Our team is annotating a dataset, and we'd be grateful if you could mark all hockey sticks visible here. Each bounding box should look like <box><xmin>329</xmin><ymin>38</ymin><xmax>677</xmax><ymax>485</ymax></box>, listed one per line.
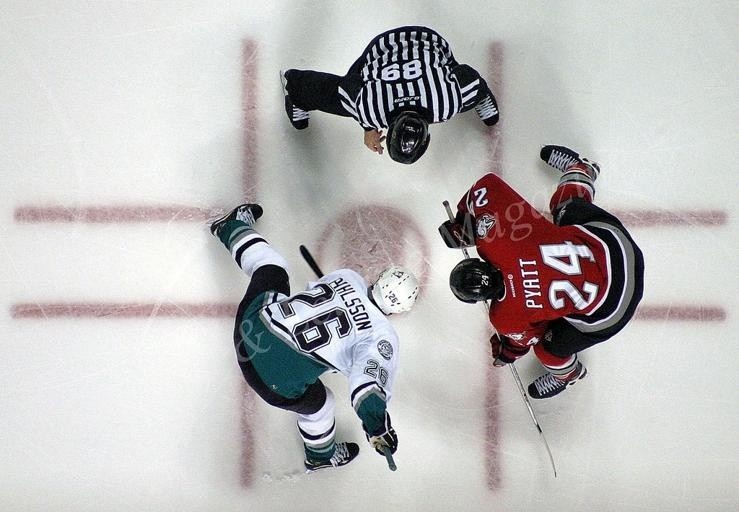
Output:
<box><xmin>443</xmin><ymin>200</ymin><xmax>556</xmax><ymax>477</ymax></box>
<box><xmin>299</xmin><ymin>245</ymin><xmax>397</xmax><ymax>471</ymax></box>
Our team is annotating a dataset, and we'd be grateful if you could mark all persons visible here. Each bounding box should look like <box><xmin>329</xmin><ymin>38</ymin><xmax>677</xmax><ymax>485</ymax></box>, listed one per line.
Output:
<box><xmin>282</xmin><ymin>25</ymin><xmax>501</xmax><ymax>164</ymax></box>
<box><xmin>438</xmin><ymin>143</ymin><xmax>646</xmax><ymax>400</ymax></box>
<box><xmin>209</xmin><ymin>202</ymin><xmax>423</xmax><ymax>474</ymax></box>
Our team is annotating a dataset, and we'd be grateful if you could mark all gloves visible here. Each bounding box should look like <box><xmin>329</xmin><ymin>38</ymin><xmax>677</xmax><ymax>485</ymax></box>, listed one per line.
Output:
<box><xmin>438</xmin><ymin>218</ymin><xmax>475</xmax><ymax>251</ymax></box>
<box><xmin>359</xmin><ymin>407</ymin><xmax>400</xmax><ymax>457</ymax></box>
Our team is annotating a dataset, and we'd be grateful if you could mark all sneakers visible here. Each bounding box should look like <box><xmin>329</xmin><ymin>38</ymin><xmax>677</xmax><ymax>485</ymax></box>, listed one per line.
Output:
<box><xmin>282</xmin><ymin>92</ymin><xmax>309</xmax><ymax>132</ymax></box>
<box><xmin>527</xmin><ymin>360</ymin><xmax>588</xmax><ymax>399</ymax></box>
<box><xmin>472</xmin><ymin>86</ymin><xmax>500</xmax><ymax>127</ymax></box>
<box><xmin>538</xmin><ymin>143</ymin><xmax>600</xmax><ymax>182</ymax></box>
<box><xmin>299</xmin><ymin>441</ymin><xmax>360</xmax><ymax>473</ymax></box>
<box><xmin>209</xmin><ymin>201</ymin><xmax>265</xmax><ymax>241</ymax></box>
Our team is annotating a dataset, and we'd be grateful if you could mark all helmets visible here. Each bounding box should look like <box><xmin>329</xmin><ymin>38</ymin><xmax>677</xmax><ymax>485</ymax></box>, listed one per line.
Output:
<box><xmin>448</xmin><ymin>256</ymin><xmax>504</xmax><ymax>305</ymax></box>
<box><xmin>381</xmin><ymin>110</ymin><xmax>431</xmax><ymax>167</ymax></box>
<box><xmin>368</xmin><ymin>262</ymin><xmax>422</xmax><ymax>315</ymax></box>
